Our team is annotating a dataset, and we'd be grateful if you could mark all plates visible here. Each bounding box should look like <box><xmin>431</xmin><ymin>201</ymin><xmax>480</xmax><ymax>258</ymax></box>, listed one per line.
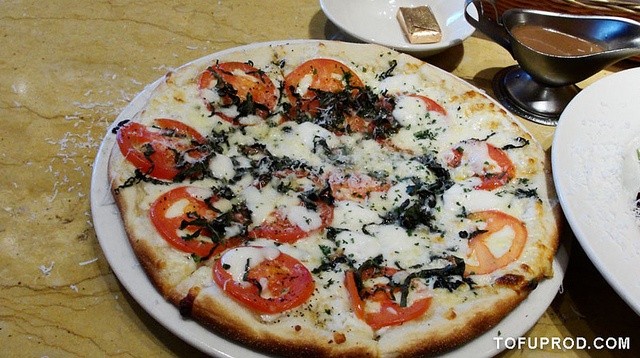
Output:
<box><xmin>551</xmin><ymin>66</ymin><xmax>640</xmax><ymax>319</ymax></box>
<box><xmin>318</xmin><ymin>0</ymin><xmax>479</xmax><ymax>57</ymax></box>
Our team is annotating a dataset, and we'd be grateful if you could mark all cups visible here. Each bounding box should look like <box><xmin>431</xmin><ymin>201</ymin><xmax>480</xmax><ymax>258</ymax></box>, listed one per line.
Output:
<box><xmin>464</xmin><ymin>0</ymin><xmax>639</xmax><ymax>126</ymax></box>
